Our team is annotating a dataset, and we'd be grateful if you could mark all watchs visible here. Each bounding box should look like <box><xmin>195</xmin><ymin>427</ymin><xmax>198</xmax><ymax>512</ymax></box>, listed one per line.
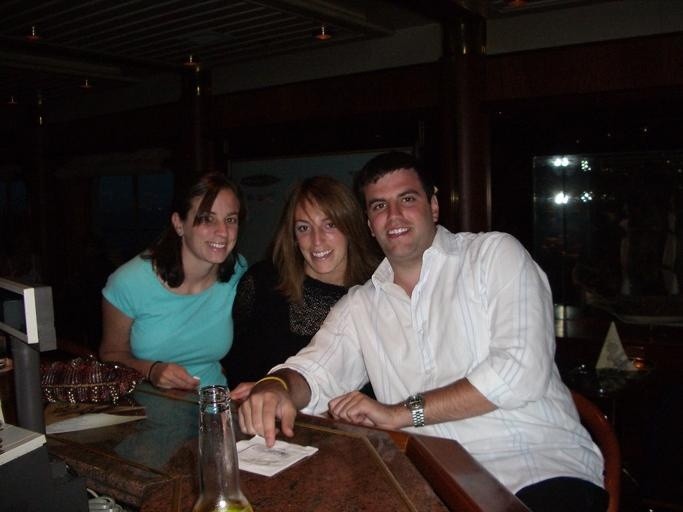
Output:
<box><xmin>407</xmin><ymin>391</ymin><xmax>427</xmax><ymax>426</ymax></box>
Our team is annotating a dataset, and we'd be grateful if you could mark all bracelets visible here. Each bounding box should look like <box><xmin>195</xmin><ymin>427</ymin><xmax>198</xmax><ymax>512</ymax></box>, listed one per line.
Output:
<box><xmin>253</xmin><ymin>375</ymin><xmax>291</xmax><ymax>392</ymax></box>
<box><xmin>146</xmin><ymin>361</ymin><xmax>162</xmax><ymax>383</ymax></box>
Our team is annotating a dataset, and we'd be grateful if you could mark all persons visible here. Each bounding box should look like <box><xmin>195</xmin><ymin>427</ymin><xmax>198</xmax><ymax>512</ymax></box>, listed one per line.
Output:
<box><xmin>97</xmin><ymin>168</ymin><xmax>250</xmax><ymax>394</ymax></box>
<box><xmin>236</xmin><ymin>151</ymin><xmax>611</xmax><ymax>511</ymax></box>
<box><xmin>218</xmin><ymin>175</ymin><xmax>380</xmax><ymax>402</ymax></box>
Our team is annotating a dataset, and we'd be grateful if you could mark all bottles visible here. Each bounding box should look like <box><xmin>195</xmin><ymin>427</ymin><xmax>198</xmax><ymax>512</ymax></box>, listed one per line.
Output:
<box><xmin>190</xmin><ymin>384</ymin><xmax>251</xmax><ymax>512</ymax></box>
